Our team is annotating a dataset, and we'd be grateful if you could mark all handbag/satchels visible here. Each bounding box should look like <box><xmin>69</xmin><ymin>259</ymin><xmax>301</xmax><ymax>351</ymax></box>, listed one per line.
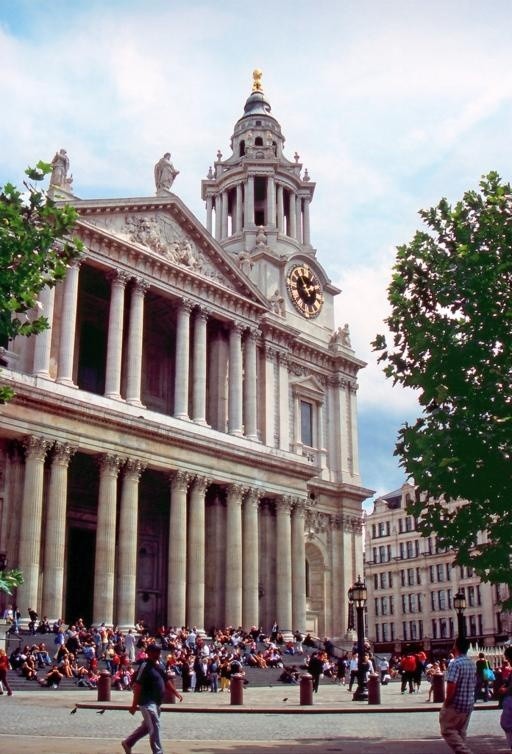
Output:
<box><xmin>483</xmin><ymin>669</ymin><xmax>496</xmax><ymax>681</ymax></box>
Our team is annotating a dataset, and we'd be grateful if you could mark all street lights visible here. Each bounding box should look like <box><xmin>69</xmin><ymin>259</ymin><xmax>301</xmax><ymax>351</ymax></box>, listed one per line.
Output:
<box><xmin>348</xmin><ymin>573</ymin><xmax>370</xmax><ymax>702</ymax></box>
<box><xmin>453</xmin><ymin>586</ymin><xmax>467</xmax><ymax>639</ymax></box>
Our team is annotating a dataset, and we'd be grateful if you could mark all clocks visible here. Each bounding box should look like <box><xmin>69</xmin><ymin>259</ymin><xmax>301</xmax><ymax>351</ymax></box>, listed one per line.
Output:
<box><xmin>287</xmin><ymin>263</ymin><xmax>325</xmax><ymax>319</ymax></box>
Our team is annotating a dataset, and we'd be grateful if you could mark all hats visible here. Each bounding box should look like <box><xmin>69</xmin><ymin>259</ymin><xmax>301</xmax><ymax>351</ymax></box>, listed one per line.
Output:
<box><xmin>479</xmin><ymin>653</ymin><xmax>484</xmax><ymax>658</ymax></box>
<box><xmin>145</xmin><ymin>645</ymin><xmax>164</xmax><ymax>652</ymax></box>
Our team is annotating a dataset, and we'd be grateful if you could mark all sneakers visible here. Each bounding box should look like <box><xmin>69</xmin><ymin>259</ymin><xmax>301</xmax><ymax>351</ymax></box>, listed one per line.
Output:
<box><xmin>122</xmin><ymin>741</ymin><xmax>131</xmax><ymax>754</ymax></box>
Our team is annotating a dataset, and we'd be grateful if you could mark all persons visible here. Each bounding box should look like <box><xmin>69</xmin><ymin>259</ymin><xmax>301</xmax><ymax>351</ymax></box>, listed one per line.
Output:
<box><xmin>121</xmin><ymin>644</ymin><xmax>186</xmax><ymax>753</ymax></box>
<box><xmin>495</xmin><ymin>644</ymin><xmax>512</xmax><ymax>751</ymax></box>
<box><xmin>437</xmin><ymin>636</ymin><xmax>478</xmax><ymax>753</ymax></box>
<box><xmin>139</xmin><ymin>216</ymin><xmax>208</xmax><ymax>274</ymax></box>
<box><xmin>0</xmin><ymin>606</ymin><xmax>512</xmax><ymax>701</ymax></box>
<box><xmin>49</xmin><ymin>148</ymin><xmax>71</xmax><ymax>186</ymax></box>
<box><xmin>158</xmin><ymin>151</ymin><xmax>178</xmax><ymax>188</ymax></box>
<box><xmin>330</xmin><ymin>323</ymin><xmax>351</xmax><ymax>347</ymax></box>
